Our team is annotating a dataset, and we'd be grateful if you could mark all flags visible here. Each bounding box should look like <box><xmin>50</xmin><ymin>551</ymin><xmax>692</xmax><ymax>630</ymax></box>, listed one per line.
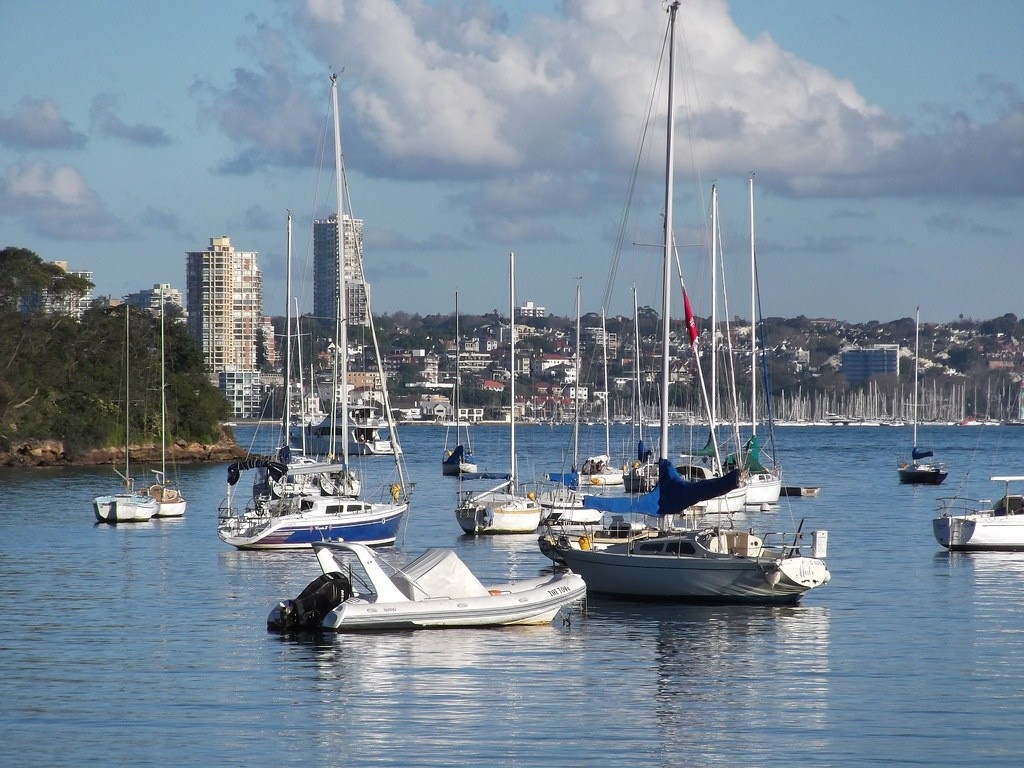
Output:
<box><xmin>684</xmin><ymin>290</ymin><xmax>699</xmax><ymax>349</ymax></box>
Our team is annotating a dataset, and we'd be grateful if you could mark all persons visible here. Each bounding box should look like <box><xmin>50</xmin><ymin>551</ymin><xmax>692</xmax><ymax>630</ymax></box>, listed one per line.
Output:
<box><xmin>581</xmin><ymin>459</ymin><xmax>606</xmax><ymax>475</ymax></box>
<box><xmin>359</xmin><ymin>434</ymin><xmax>364</xmax><ymax>442</ymax></box>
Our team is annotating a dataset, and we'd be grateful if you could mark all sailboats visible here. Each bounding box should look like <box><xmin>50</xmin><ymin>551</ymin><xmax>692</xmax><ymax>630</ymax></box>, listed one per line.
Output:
<box><xmin>443</xmin><ymin>291</ymin><xmax>476</xmax><ymax>477</ymax></box>
<box><xmin>140</xmin><ymin>286</ymin><xmax>188</xmax><ymax>517</ymax></box>
<box><xmin>624</xmin><ymin>282</ymin><xmax>661</xmax><ymax>492</ymax></box>
<box><xmin>250</xmin><ymin>209</ymin><xmax>359</xmax><ymax>513</ymax></box>
<box><xmin>931</xmin><ymin>474</ymin><xmax>1024</xmax><ymax>549</ymax></box>
<box><xmin>454</xmin><ymin>252</ymin><xmax>541</xmax><ymax>535</ymax></box>
<box><xmin>729</xmin><ymin>173</ymin><xmax>784</xmax><ymax>505</ymax></box>
<box><xmin>93</xmin><ymin>305</ymin><xmax>158</xmax><ymax>522</ymax></box>
<box><xmin>539</xmin><ymin>0</ymin><xmax>832</xmax><ymax>603</ymax></box>
<box><xmin>897</xmin><ymin>304</ymin><xmax>950</xmax><ymax>484</ymax></box>
<box><xmin>669</xmin><ymin>182</ymin><xmax>747</xmax><ymax>514</ymax></box>
<box><xmin>216</xmin><ymin>71</ymin><xmax>411</xmax><ymax>550</ymax></box>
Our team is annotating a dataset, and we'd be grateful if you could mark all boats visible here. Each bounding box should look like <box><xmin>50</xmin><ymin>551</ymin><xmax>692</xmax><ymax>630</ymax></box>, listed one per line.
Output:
<box><xmin>529</xmin><ymin>486</ymin><xmax>605</xmax><ymax>523</ymax></box>
<box><xmin>568</xmin><ymin>308</ymin><xmax>627</xmax><ymax>486</ymax></box>
<box><xmin>270</xmin><ymin>536</ymin><xmax>586</xmax><ymax>638</ymax></box>
